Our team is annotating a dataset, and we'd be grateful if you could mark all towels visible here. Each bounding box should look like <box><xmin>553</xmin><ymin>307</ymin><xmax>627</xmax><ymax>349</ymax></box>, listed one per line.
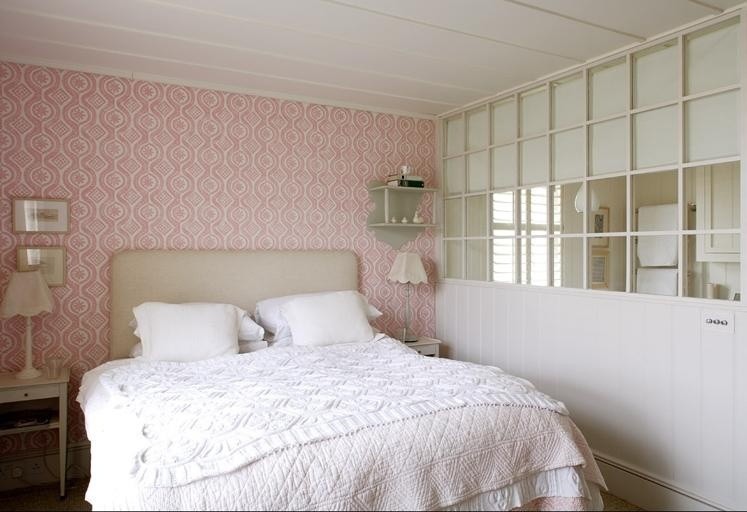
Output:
<box><xmin>637</xmin><ymin>199</ymin><xmax>681</xmax><ymax>268</ymax></box>
<box><xmin>636</xmin><ymin>268</ymin><xmax>679</xmax><ymax>297</ymax></box>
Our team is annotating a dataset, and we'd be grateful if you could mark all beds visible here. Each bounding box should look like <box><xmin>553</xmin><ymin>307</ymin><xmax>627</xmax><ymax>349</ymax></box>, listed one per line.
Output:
<box><xmin>78</xmin><ymin>249</ymin><xmax>608</xmax><ymax>512</ymax></box>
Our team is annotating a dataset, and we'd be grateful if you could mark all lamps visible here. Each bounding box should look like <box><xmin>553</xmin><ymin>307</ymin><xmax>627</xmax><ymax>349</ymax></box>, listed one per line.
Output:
<box><xmin>0</xmin><ymin>271</ymin><xmax>56</xmax><ymax>379</ymax></box>
<box><xmin>388</xmin><ymin>252</ymin><xmax>429</xmax><ymax>342</ymax></box>
<box><xmin>574</xmin><ymin>181</ymin><xmax>598</xmax><ymax>213</ymax></box>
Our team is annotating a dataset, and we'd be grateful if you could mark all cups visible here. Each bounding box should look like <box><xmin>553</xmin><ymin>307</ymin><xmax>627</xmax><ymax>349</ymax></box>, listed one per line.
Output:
<box><xmin>705</xmin><ymin>283</ymin><xmax>719</xmax><ymax>299</ymax></box>
<box><xmin>46</xmin><ymin>359</ymin><xmax>60</xmax><ymax>378</ymax></box>
<box><xmin>395</xmin><ymin>327</ymin><xmax>406</xmax><ymax>344</ymax></box>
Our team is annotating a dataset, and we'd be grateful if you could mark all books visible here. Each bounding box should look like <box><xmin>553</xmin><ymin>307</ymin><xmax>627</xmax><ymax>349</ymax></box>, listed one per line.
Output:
<box><xmin>387</xmin><ymin>174</ymin><xmax>424</xmax><ymax>188</ymax></box>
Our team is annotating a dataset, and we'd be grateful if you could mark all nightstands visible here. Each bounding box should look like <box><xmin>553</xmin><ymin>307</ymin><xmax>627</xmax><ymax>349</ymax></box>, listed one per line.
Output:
<box><xmin>404</xmin><ymin>336</ymin><xmax>441</xmax><ymax>358</ymax></box>
<box><xmin>0</xmin><ymin>368</ymin><xmax>71</xmax><ymax>498</ymax></box>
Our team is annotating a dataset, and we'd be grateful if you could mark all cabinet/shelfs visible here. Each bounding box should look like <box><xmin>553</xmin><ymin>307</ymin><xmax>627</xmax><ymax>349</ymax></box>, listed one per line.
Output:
<box><xmin>694</xmin><ymin>159</ymin><xmax>742</xmax><ymax>263</ymax></box>
<box><xmin>367</xmin><ymin>185</ymin><xmax>438</xmax><ymax>230</ymax></box>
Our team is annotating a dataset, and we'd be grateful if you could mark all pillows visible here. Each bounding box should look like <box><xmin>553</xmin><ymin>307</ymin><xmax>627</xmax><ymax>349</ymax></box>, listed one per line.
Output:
<box><xmin>254</xmin><ymin>289</ymin><xmax>384</xmax><ymax>346</ymax></box>
<box><xmin>278</xmin><ymin>293</ymin><xmax>376</xmax><ymax>343</ymax></box>
<box><xmin>135</xmin><ymin>301</ymin><xmax>241</xmax><ymax>357</ymax></box>
<box><xmin>130</xmin><ymin>299</ymin><xmax>267</xmax><ymax>359</ymax></box>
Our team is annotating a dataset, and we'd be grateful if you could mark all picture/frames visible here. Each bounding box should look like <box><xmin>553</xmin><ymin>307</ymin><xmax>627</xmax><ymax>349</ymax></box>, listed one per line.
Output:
<box><xmin>11</xmin><ymin>197</ymin><xmax>72</xmax><ymax>235</ymax></box>
<box><xmin>15</xmin><ymin>244</ymin><xmax>67</xmax><ymax>287</ymax></box>
<box><xmin>588</xmin><ymin>251</ymin><xmax>610</xmax><ymax>288</ymax></box>
<box><xmin>591</xmin><ymin>206</ymin><xmax>610</xmax><ymax>249</ymax></box>
